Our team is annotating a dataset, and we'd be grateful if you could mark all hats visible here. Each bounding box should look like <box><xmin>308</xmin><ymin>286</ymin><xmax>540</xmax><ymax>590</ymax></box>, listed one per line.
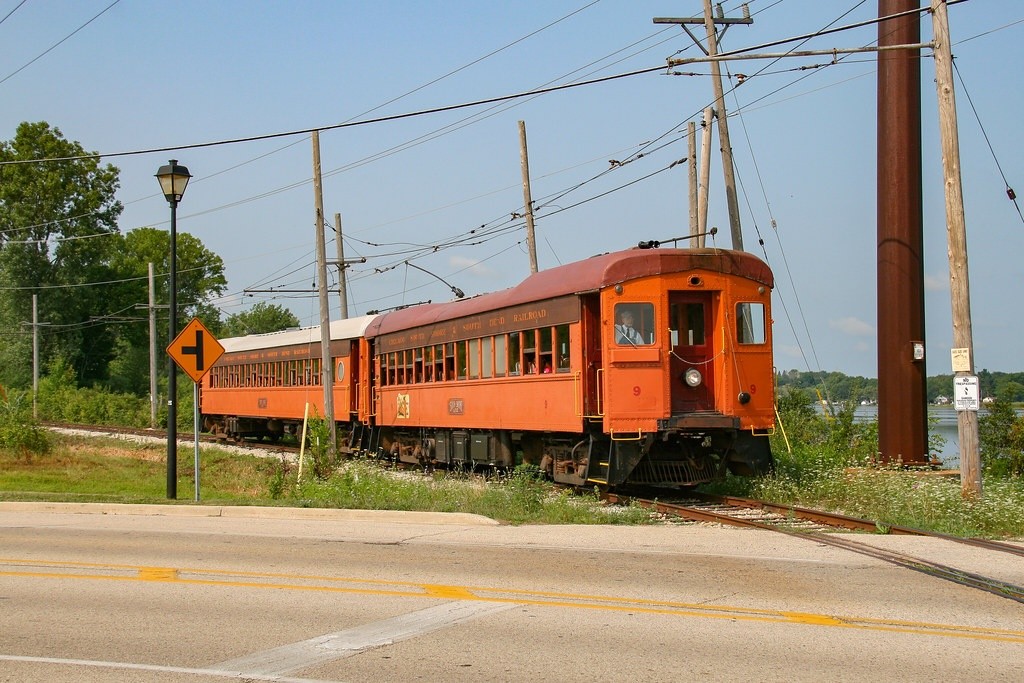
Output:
<box><xmin>621</xmin><ymin>311</ymin><xmax>635</xmax><ymax>319</ymax></box>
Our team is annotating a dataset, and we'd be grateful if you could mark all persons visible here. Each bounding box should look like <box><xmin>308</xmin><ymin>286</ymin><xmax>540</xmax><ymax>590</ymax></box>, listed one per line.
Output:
<box><xmin>614</xmin><ymin>310</ymin><xmax>645</xmax><ymax>344</ymax></box>
<box><xmin>532</xmin><ymin>356</ymin><xmax>552</xmax><ymax>374</ymax></box>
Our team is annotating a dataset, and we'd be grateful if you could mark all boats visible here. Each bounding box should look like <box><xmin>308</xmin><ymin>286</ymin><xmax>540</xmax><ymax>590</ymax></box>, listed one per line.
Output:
<box><xmin>861</xmin><ymin>400</ymin><xmax>875</xmax><ymax>406</ymax></box>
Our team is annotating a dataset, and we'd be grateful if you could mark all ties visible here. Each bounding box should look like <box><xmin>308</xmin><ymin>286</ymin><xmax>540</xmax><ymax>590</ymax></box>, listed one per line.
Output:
<box><xmin>626</xmin><ymin>328</ymin><xmax>630</xmax><ymax>337</ymax></box>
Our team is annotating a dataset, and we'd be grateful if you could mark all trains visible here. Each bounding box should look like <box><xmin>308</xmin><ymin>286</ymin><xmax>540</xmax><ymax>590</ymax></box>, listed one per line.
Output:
<box><xmin>196</xmin><ymin>229</ymin><xmax>779</xmax><ymax>494</ymax></box>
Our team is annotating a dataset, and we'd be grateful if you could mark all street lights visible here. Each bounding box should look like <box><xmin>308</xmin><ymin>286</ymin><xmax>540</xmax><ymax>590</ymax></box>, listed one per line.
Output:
<box><xmin>155</xmin><ymin>156</ymin><xmax>194</xmax><ymax>499</ymax></box>
